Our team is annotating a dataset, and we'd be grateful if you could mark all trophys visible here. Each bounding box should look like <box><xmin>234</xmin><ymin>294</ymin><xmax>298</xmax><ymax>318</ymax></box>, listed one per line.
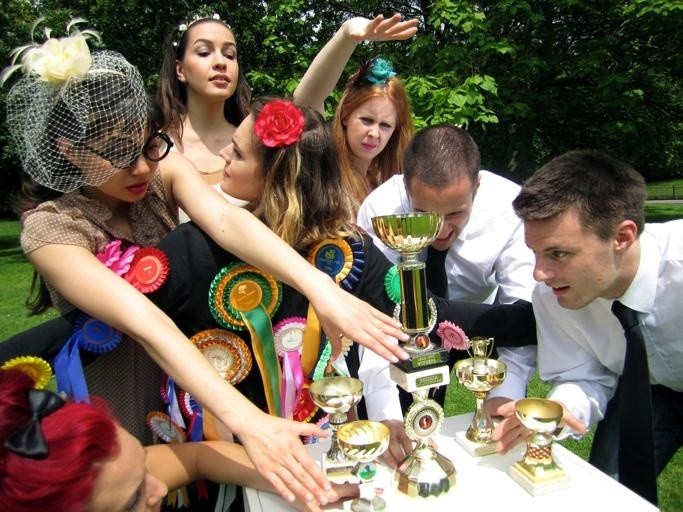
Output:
<box><xmin>338</xmin><ymin>420</ymin><xmax>389</xmax><ymax>511</ymax></box>
<box><xmin>309</xmin><ymin>377</ymin><xmax>363</xmax><ymax>429</ymax></box>
<box><xmin>453</xmin><ymin>335</ymin><xmax>507</xmax><ymax>456</ymax></box>
<box><xmin>371</xmin><ymin>213</ymin><xmax>451</xmax><ymax>394</ymax></box>
<box><xmin>395</xmin><ymin>400</ymin><xmax>456</xmax><ymax>497</ymax></box>
<box><xmin>510</xmin><ymin>398</ymin><xmax>567</xmax><ymax>496</ymax></box>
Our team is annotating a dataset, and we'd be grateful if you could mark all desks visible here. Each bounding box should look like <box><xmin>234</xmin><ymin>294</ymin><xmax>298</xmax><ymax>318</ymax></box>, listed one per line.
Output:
<box><xmin>241</xmin><ymin>410</ymin><xmax>660</xmax><ymax>511</ymax></box>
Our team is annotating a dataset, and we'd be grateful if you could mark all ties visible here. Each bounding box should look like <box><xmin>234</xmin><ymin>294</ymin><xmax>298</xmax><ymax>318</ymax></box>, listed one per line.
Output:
<box><xmin>611</xmin><ymin>301</ymin><xmax>658</xmax><ymax>506</ymax></box>
<box><xmin>425</xmin><ymin>245</ymin><xmax>451</xmax><ymax>298</ymax></box>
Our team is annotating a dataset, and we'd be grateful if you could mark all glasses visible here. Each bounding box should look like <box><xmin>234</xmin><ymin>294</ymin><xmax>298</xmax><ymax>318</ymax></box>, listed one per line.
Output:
<box><xmin>75</xmin><ymin>133</ymin><xmax>174</xmax><ymax>168</ymax></box>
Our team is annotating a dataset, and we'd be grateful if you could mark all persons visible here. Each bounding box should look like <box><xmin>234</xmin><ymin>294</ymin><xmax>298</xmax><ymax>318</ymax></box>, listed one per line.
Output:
<box><xmin>358</xmin><ymin>122</ymin><xmax>538</xmax><ymax>423</ymax></box>
<box><xmin>331</xmin><ymin>55</ymin><xmax>414</xmax><ymax>209</ymax></box>
<box><xmin>0</xmin><ymin>365</ymin><xmax>360</xmax><ymax>512</ymax></box>
<box><xmin>157</xmin><ymin>12</ymin><xmax>418</xmax><ymax>222</ymax></box>
<box><xmin>493</xmin><ymin>149</ymin><xmax>682</xmax><ymax>485</ymax></box>
<box><xmin>0</xmin><ymin>93</ymin><xmax>538</xmax><ymax>433</ymax></box>
<box><xmin>0</xmin><ymin>17</ymin><xmax>410</xmax><ymax>506</ymax></box>
